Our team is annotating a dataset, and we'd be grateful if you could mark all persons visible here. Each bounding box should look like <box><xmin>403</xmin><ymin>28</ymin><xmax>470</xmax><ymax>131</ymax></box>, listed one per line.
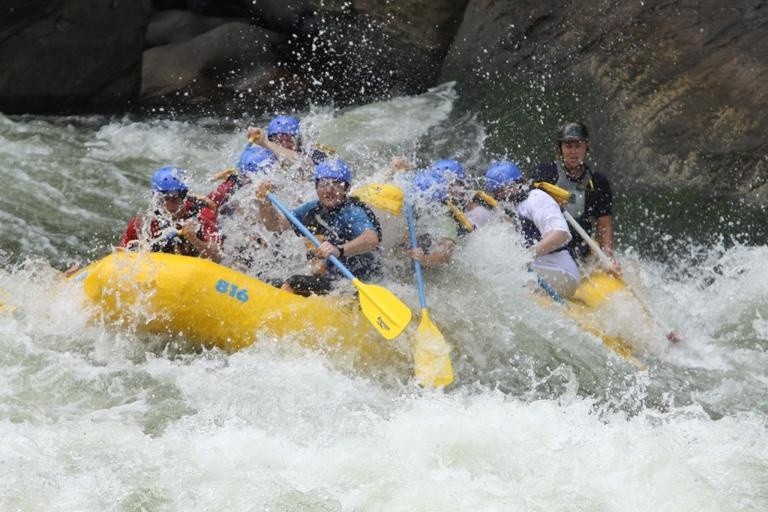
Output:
<box><xmin>370</xmin><ymin>120</ymin><xmax>489</xmax><ymax>284</ymax></box>
<box><xmin>484</xmin><ymin>161</ymin><xmax>579</xmax><ymax>300</ymax></box>
<box><xmin>120</xmin><ymin>115</ymin><xmax>383</xmax><ymax>299</ymax></box>
<box><xmin>523</xmin><ymin>122</ymin><xmax>623</xmax><ymax>281</ymax></box>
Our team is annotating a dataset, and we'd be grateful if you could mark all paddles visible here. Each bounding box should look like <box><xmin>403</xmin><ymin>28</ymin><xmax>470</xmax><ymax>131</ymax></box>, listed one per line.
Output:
<box><xmin>264</xmin><ymin>189</ymin><xmax>411</xmax><ymax>340</ymax></box>
<box><xmin>402</xmin><ymin>169</ymin><xmax>453</xmax><ymax>389</ymax></box>
<box><xmin>473</xmin><ymin>192</ymin><xmax>631</xmax><ymax>361</ymax></box>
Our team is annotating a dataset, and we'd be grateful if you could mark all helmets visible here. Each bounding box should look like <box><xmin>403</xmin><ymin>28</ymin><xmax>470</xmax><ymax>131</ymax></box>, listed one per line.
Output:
<box><xmin>268</xmin><ymin>115</ymin><xmax>301</xmax><ymax>139</ymax></box>
<box><xmin>413</xmin><ymin>172</ymin><xmax>449</xmax><ymax>204</ymax></box>
<box><xmin>433</xmin><ymin>159</ymin><xmax>466</xmax><ymax>185</ymax></box>
<box><xmin>314</xmin><ymin>158</ymin><xmax>351</xmax><ymax>185</ymax></box>
<box><xmin>238</xmin><ymin>147</ymin><xmax>279</xmax><ymax>177</ymax></box>
<box><xmin>485</xmin><ymin>160</ymin><xmax>522</xmax><ymax>191</ymax></box>
<box><xmin>152</xmin><ymin>167</ymin><xmax>190</xmax><ymax>193</ymax></box>
<box><xmin>555</xmin><ymin>121</ymin><xmax>589</xmax><ymax>152</ymax></box>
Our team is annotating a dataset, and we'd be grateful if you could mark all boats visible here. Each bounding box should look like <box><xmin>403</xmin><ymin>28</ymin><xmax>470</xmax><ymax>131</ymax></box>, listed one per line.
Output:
<box><xmin>58</xmin><ymin>181</ymin><xmax>671</xmax><ymax>390</ymax></box>
